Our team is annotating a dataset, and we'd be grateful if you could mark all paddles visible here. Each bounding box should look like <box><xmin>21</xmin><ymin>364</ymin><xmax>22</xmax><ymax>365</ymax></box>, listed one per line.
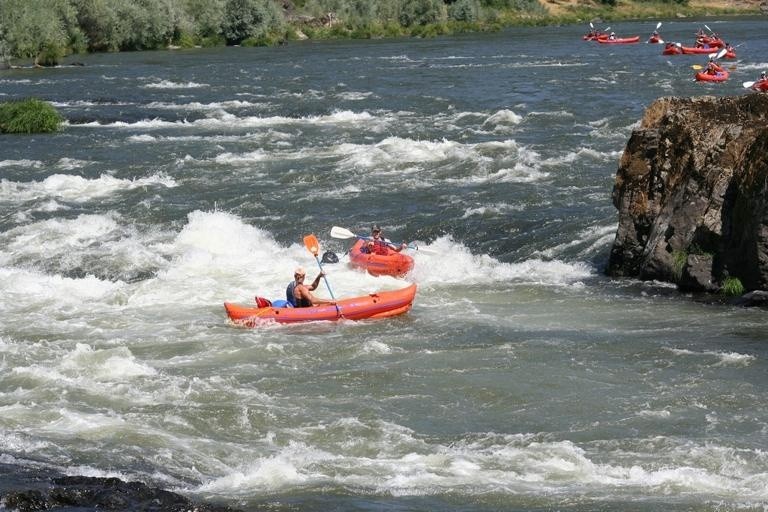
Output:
<box><xmin>590</xmin><ymin>23</ymin><xmax>594</xmax><ymax>29</ymax></box>
<box><xmin>604</xmin><ymin>27</ymin><xmax>611</xmax><ymax>32</ymax></box>
<box><xmin>303</xmin><ymin>234</ymin><xmax>344</xmax><ymax>319</ymax></box>
<box><xmin>330</xmin><ymin>226</ymin><xmax>438</xmax><ymax>256</ymax></box>
<box><xmin>705</xmin><ymin>25</ymin><xmax>714</xmax><ymax>34</ymax></box>
<box><xmin>708</xmin><ymin>44</ymin><xmax>743</xmax><ymax>59</ymax></box>
<box><xmin>643</xmin><ymin>22</ymin><xmax>662</xmax><ymax>43</ymax></box>
<box><xmin>693</xmin><ymin>64</ymin><xmax>737</xmax><ymax>71</ymax></box>
<box><xmin>692</xmin><ymin>48</ymin><xmax>727</xmax><ymax>82</ymax></box>
<box><xmin>742</xmin><ymin>81</ymin><xmax>756</xmax><ymax>89</ymax></box>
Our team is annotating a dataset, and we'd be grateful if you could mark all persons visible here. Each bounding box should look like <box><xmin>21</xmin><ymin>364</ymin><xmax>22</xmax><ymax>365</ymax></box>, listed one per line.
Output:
<box><xmin>587</xmin><ymin>28</ymin><xmax>734</xmax><ymax>75</ymax></box>
<box><xmin>362</xmin><ymin>223</ymin><xmax>406</xmax><ymax>254</ymax></box>
<box><xmin>286</xmin><ymin>268</ymin><xmax>335</xmax><ymax>306</ymax></box>
<box><xmin>760</xmin><ymin>71</ymin><xmax>768</xmax><ymax>81</ymax></box>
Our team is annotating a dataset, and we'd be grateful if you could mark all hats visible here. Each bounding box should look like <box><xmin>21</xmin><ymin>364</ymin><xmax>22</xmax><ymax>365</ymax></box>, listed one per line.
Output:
<box><xmin>295</xmin><ymin>266</ymin><xmax>307</xmax><ymax>274</ymax></box>
<box><xmin>372</xmin><ymin>224</ymin><xmax>380</xmax><ymax>232</ymax></box>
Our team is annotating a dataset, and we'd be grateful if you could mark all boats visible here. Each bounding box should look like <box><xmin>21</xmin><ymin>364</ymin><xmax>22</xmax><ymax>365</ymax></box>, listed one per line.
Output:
<box><xmin>650</xmin><ymin>34</ymin><xmax>661</xmax><ymax>43</ymax></box>
<box><xmin>349</xmin><ymin>237</ymin><xmax>416</xmax><ymax>278</ymax></box>
<box><xmin>598</xmin><ymin>36</ymin><xmax>640</xmax><ymax>44</ymax></box>
<box><xmin>695</xmin><ymin>71</ymin><xmax>730</xmax><ymax>82</ymax></box>
<box><xmin>748</xmin><ymin>79</ymin><xmax>768</xmax><ymax>93</ymax></box>
<box><xmin>224</xmin><ymin>281</ymin><xmax>418</xmax><ymax>329</ymax></box>
<box><xmin>663</xmin><ymin>46</ymin><xmax>681</xmax><ymax>56</ymax></box>
<box><xmin>724</xmin><ymin>50</ymin><xmax>736</xmax><ymax>59</ymax></box>
<box><xmin>681</xmin><ymin>38</ymin><xmax>726</xmax><ymax>55</ymax></box>
<box><xmin>582</xmin><ymin>33</ymin><xmax>609</xmax><ymax>42</ymax></box>
